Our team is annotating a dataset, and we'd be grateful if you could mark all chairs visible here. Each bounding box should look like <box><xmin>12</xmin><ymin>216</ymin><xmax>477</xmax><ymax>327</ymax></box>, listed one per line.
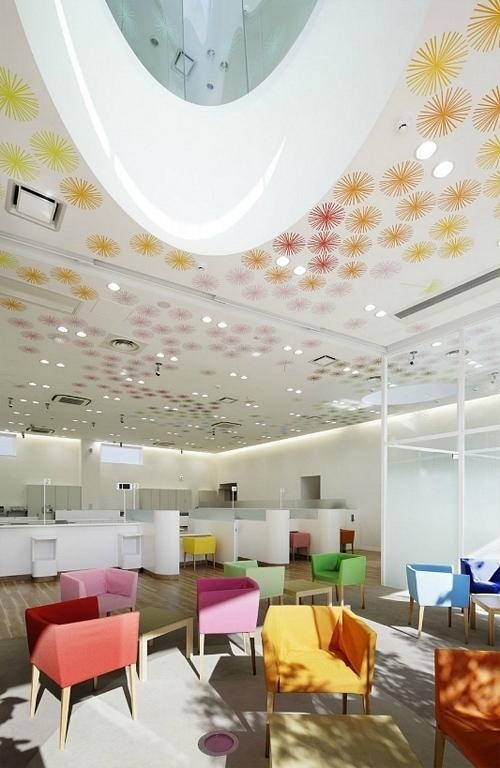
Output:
<box><xmin>196</xmin><ymin>577</ymin><xmax>260</xmax><ymax>656</ymax></box>
<box><xmin>24</xmin><ymin>596</ymin><xmax>140</xmax><ymax>751</ymax></box>
<box><xmin>224</xmin><ymin>560</ymin><xmax>285</xmax><ymax>605</ymax></box>
<box><xmin>460</xmin><ymin>558</ymin><xmax>500</xmax><ymax>593</ymax></box>
<box><xmin>434</xmin><ymin>647</ymin><xmax>500</xmax><ymax>768</ymax></box>
<box><xmin>406</xmin><ymin>563</ymin><xmax>471</xmax><ymax>644</ymax></box>
<box><xmin>261</xmin><ymin>605</ymin><xmax>377</xmax><ymax>715</ymax></box>
<box><xmin>60</xmin><ymin>568</ymin><xmax>138</xmax><ymax>616</ymax></box>
<box><xmin>311</xmin><ymin>552</ymin><xmax>366</xmax><ymax>609</ymax></box>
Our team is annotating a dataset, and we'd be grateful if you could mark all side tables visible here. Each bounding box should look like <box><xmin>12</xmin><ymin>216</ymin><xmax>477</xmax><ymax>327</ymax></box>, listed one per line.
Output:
<box><xmin>136</xmin><ymin>607</ymin><xmax>195</xmax><ymax>682</ymax></box>
<box><xmin>283</xmin><ymin>579</ymin><xmax>332</xmax><ymax>606</ymax></box>
<box><xmin>265</xmin><ymin>713</ymin><xmax>424</xmax><ymax>768</ymax></box>
<box><xmin>470</xmin><ymin>594</ymin><xmax>500</xmax><ymax>646</ymax></box>
